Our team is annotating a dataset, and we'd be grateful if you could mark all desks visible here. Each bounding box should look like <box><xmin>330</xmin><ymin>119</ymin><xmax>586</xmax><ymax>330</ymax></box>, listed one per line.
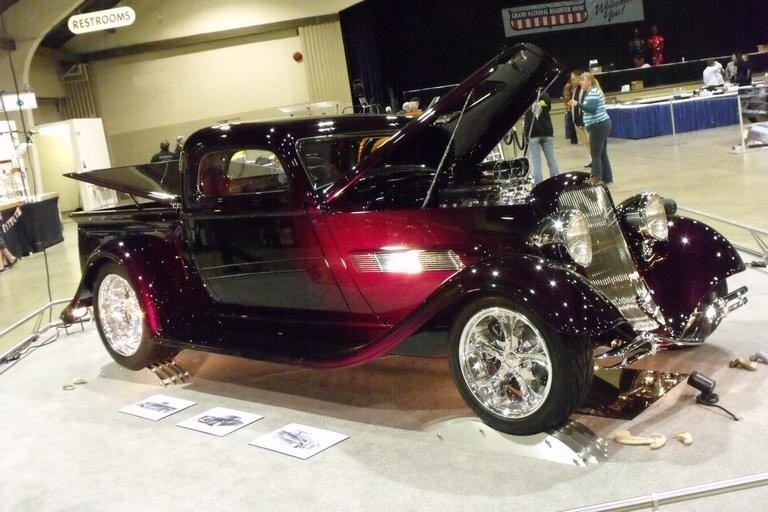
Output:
<box><xmin>593</xmin><ymin>50</ymin><xmax>768</xmax><ymax>139</ymax></box>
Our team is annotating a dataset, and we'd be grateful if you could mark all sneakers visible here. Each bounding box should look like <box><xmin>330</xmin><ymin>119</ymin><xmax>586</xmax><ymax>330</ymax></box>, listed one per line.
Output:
<box><xmin>582</xmin><ymin>175</ymin><xmax>613</xmax><ymax>186</ymax></box>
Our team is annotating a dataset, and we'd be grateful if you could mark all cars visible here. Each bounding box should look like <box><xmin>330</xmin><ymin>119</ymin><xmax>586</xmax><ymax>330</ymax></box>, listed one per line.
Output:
<box><xmin>56</xmin><ymin>38</ymin><xmax>749</xmax><ymax>438</ymax></box>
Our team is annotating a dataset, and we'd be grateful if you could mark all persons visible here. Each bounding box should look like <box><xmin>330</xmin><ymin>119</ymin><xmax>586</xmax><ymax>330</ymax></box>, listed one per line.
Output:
<box><xmin>737</xmin><ymin>53</ymin><xmax>753</xmax><ymax>86</ymax></box>
<box><xmin>571</xmin><ymin>70</ymin><xmax>593</xmax><ymax>167</ymax></box>
<box><xmin>524</xmin><ymin>92</ymin><xmax>560</xmax><ymax>187</ymax></box>
<box><xmin>395</xmin><ymin>97</ymin><xmax>422</xmax><ymax>116</ymax></box>
<box><xmin>567</xmin><ymin>72</ymin><xmax>613</xmax><ymax>184</ymax></box>
<box><xmin>150</xmin><ymin>140</ymin><xmax>179</xmax><ymax>162</ymax></box>
<box><xmin>563</xmin><ymin>78</ymin><xmax>578</xmax><ymax>138</ymax></box>
<box><xmin>633</xmin><ymin>54</ymin><xmax>652</xmax><ymax>68</ymax></box>
<box><xmin>174</xmin><ymin>135</ymin><xmax>184</xmax><ymax>155</ymax></box>
<box><xmin>726</xmin><ymin>54</ymin><xmax>741</xmax><ymax>83</ymax></box>
<box><xmin>703</xmin><ymin>59</ymin><xmax>726</xmax><ymax>89</ymax></box>
